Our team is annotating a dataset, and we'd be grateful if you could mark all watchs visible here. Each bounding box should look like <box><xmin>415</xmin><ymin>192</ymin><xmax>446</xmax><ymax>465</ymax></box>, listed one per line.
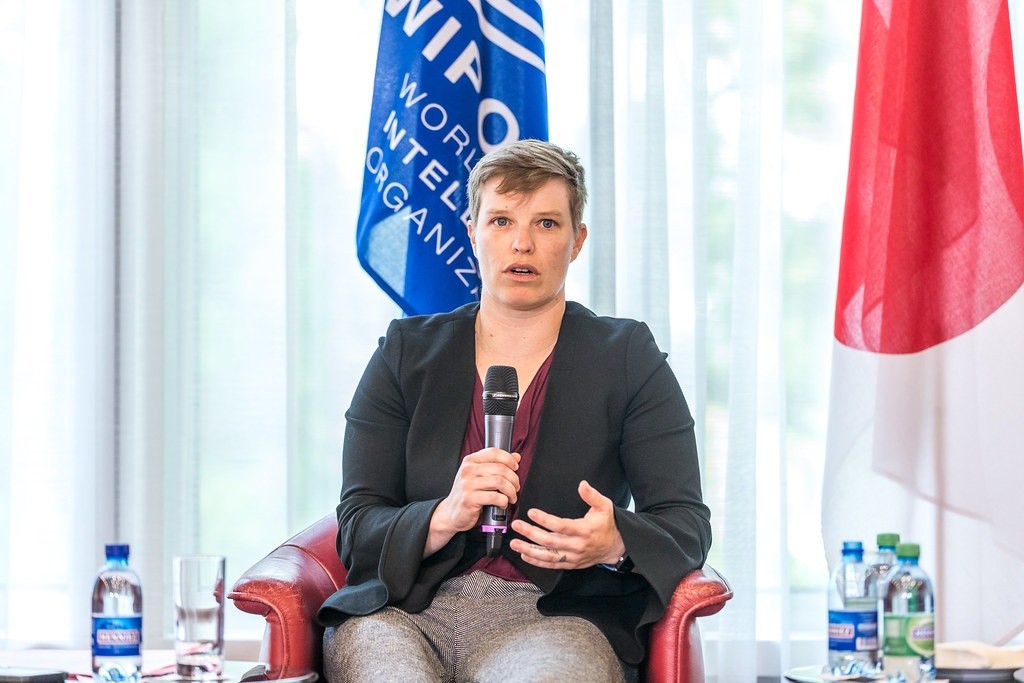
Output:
<box><xmin>597</xmin><ymin>551</ymin><xmax>634</xmax><ymax>574</ymax></box>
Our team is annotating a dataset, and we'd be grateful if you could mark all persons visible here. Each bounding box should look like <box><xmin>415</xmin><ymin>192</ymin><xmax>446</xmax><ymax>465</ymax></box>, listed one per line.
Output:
<box><xmin>315</xmin><ymin>140</ymin><xmax>712</xmax><ymax>682</ymax></box>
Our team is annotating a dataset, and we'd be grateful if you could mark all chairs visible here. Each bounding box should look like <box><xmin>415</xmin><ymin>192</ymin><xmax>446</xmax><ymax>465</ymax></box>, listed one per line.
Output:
<box><xmin>231</xmin><ymin>522</ymin><xmax>736</xmax><ymax>683</ymax></box>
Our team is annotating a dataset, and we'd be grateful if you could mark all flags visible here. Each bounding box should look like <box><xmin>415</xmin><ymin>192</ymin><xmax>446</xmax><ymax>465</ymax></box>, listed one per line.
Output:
<box><xmin>821</xmin><ymin>0</ymin><xmax>1024</xmax><ymax>645</ymax></box>
<box><xmin>357</xmin><ymin>0</ymin><xmax>550</xmax><ymax>316</ymax></box>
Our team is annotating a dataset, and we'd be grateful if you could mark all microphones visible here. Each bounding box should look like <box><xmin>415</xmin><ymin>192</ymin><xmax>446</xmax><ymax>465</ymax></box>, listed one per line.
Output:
<box><xmin>477</xmin><ymin>366</ymin><xmax>520</xmax><ymax>556</ymax></box>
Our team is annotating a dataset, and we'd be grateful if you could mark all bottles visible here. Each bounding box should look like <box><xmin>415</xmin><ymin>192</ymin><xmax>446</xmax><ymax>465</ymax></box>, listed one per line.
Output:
<box><xmin>870</xmin><ymin>533</ymin><xmax>901</xmax><ymax>601</ymax></box>
<box><xmin>90</xmin><ymin>544</ymin><xmax>144</xmax><ymax>683</ymax></box>
<box><xmin>825</xmin><ymin>539</ymin><xmax>883</xmax><ymax>679</ymax></box>
<box><xmin>879</xmin><ymin>543</ymin><xmax>937</xmax><ymax>682</ymax></box>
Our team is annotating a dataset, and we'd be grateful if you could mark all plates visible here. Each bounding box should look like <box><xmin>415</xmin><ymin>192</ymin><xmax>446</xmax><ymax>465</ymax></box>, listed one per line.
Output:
<box><xmin>929</xmin><ymin>641</ymin><xmax>1024</xmax><ymax>683</ymax></box>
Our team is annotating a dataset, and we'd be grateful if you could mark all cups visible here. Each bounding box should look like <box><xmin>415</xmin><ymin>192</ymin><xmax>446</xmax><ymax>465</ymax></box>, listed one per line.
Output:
<box><xmin>170</xmin><ymin>555</ymin><xmax>226</xmax><ymax>678</ymax></box>
